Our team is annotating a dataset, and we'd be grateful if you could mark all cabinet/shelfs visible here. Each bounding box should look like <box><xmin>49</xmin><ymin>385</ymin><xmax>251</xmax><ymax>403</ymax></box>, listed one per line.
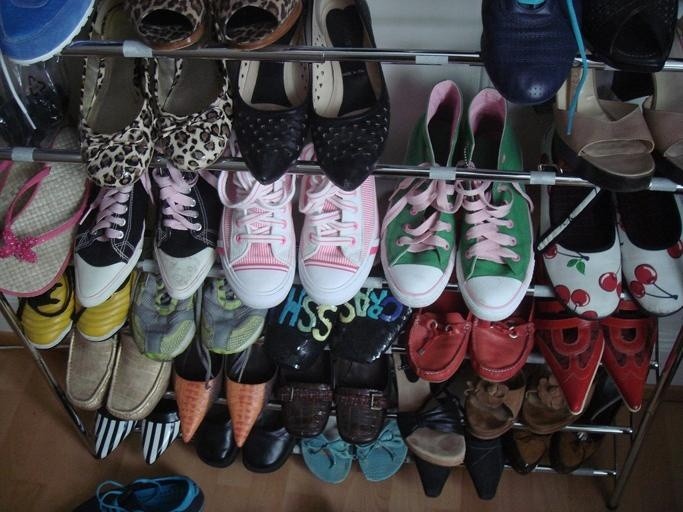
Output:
<box><xmin>0</xmin><ymin>3</ymin><xmax>683</xmax><ymax>509</ymax></box>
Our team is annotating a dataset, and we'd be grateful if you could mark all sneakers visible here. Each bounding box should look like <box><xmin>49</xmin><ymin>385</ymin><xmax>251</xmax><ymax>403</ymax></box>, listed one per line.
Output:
<box><xmin>69</xmin><ymin>475</ymin><xmax>204</xmax><ymax>510</ymax></box>
<box><xmin>0</xmin><ymin>0</ymin><xmax>683</xmax><ymax>500</ymax></box>
